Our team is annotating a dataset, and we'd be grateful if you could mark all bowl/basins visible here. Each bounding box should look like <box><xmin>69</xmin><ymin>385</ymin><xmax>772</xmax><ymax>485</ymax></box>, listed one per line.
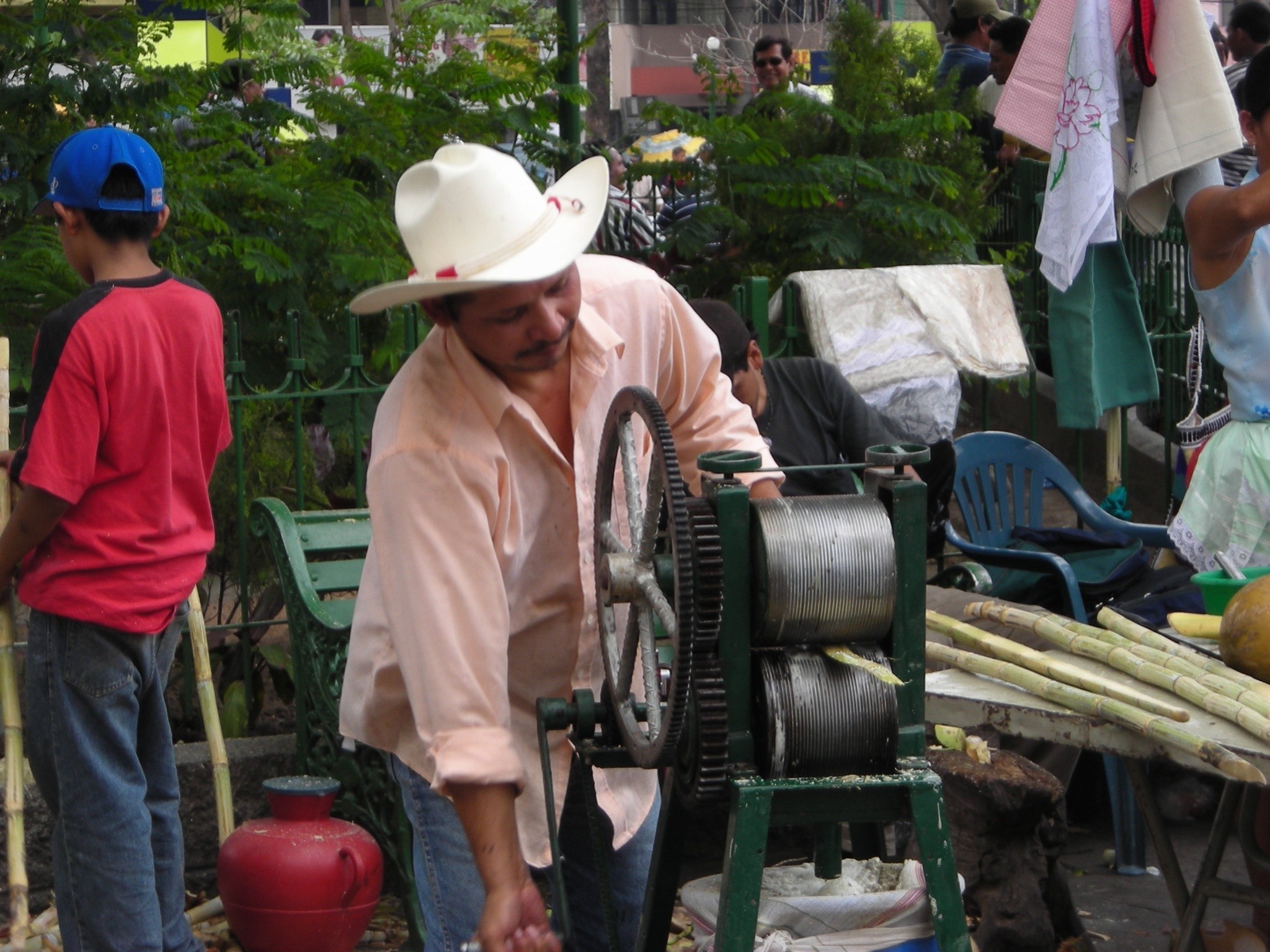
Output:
<box><xmin>1190</xmin><ymin>566</ymin><xmax>1269</xmax><ymax>616</ymax></box>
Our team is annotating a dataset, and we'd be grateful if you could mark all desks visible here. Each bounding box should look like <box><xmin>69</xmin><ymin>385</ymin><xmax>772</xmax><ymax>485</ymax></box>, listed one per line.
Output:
<box><xmin>925</xmin><ymin>626</ymin><xmax>1270</xmax><ymax>952</ymax></box>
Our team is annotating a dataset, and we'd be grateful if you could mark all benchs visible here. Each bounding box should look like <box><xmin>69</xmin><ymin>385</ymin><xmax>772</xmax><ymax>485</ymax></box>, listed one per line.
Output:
<box><xmin>252</xmin><ymin>466</ymin><xmax>996</xmax><ymax>952</ymax></box>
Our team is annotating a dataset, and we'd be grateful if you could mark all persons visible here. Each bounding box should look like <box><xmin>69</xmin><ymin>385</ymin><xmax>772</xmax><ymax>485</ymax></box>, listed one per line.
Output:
<box><xmin>0</xmin><ymin>125</ymin><xmax>232</xmax><ymax>952</ymax></box>
<box><xmin>931</xmin><ymin>0</ymin><xmax>1016</xmax><ymax>109</ymax></box>
<box><xmin>577</xmin><ymin>139</ymin><xmax>738</xmax><ymax>279</ymax></box>
<box><xmin>1212</xmin><ymin>0</ymin><xmax>1270</xmax><ymax>187</ymax></box>
<box><xmin>148</xmin><ymin>56</ymin><xmax>281</xmax><ymax>167</ymax></box>
<box><xmin>970</xmin><ymin>15</ymin><xmax>1052</xmax><ymax>163</ymax></box>
<box><xmin>749</xmin><ymin>34</ymin><xmax>830</xmax><ymax>107</ymax></box>
<box><xmin>689</xmin><ymin>295</ymin><xmax>922</xmax><ymax>496</ymax></box>
<box><xmin>339</xmin><ymin>140</ymin><xmax>790</xmax><ymax>951</ymax></box>
<box><xmin>309</xmin><ymin>28</ymin><xmax>345</xmax><ymax>86</ymax></box>
<box><xmin>439</xmin><ymin>28</ymin><xmax>477</xmax><ymax>60</ymax></box>
<box><xmin>1160</xmin><ymin>42</ymin><xmax>1269</xmax><ymax>572</ymax></box>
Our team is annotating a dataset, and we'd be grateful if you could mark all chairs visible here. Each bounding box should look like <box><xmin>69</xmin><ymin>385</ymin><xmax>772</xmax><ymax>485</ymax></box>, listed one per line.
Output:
<box><xmin>940</xmin><ymin>430</ymin><xmax>1206</xmax><ymax>877</ymax></box>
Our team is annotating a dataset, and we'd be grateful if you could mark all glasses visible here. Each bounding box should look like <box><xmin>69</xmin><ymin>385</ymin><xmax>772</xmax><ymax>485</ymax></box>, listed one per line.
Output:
<box><xmin>754</xmin><ymin>58</ymin><xmax>783</xmax><ymax>67</ymax></box>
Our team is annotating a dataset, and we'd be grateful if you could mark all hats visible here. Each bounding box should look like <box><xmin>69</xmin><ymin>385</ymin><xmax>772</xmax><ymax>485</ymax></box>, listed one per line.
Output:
<box><xmin>32</xmin><ymin>124</ymin><xmax>164</xmax><ymax>214</ymax></box>
<box><xmin>351</xmin><ymin>143</ymin><xmax>610</xmax><ymax>319</ymax></box>
<box><xmin>699</xmin><ymin>142</ymin><xmax>714</xmax><ymax>152</ymax></box>
<box><xmin>944</xmin><ymin>0</ymin><xmax>1015</xmax><ymax>35</ymax></box>
